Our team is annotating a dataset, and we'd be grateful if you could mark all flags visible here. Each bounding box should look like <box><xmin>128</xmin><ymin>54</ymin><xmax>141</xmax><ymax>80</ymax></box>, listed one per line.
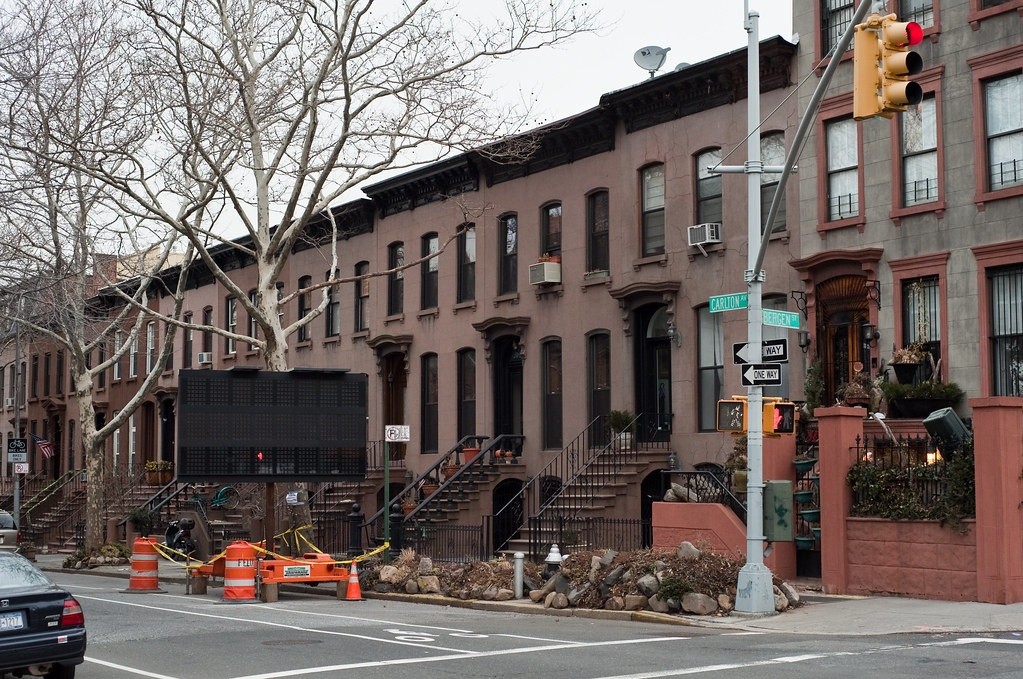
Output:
<box><xmin>29</xmin><ymin>433</ymin><xmax>54</xmax><ymax>458</ymax></box>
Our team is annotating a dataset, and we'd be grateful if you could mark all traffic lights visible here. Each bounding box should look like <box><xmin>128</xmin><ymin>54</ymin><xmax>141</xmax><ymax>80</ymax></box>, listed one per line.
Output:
<box><xmin>764</xmin><ymin>402</ymin><xmax>796</xmax><ymax>435</ymax></box>
<box><xmin>853</xmin><ymin>31</ymin><xmax>896</xmax><ymax>121</ymax></box>
<box><xmin>716</xmin><ymin>400</ymin><xmax>748</xmax><ymax>432</ymax></box>
<box><xmin>881</xmin><ymin>18</ymin><xmax>924</xmax><ymax>111</ymax></box>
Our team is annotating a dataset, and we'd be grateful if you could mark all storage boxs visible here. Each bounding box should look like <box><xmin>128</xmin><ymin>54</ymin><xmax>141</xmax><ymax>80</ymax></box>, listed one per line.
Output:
<box><xmin>180</xmin><ymin>518</ymin><xmax>195</xmax><ymax>530</ymax></box>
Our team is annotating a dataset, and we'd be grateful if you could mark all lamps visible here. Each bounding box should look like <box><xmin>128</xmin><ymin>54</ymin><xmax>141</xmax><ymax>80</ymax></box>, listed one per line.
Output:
<box><xmin>796</xmin><ymin>331</ymin><xmax>810</xmax><ymax>353</ymax></box>
<box><xmin>667</xmin><ymin>322</ymin><xmax>681</xmax><ymax>347</ymax></box>
<box><xmin>669</xmin><ymin>451</ymin><xmax>680</xmax><ymax>471</ymax></box>
<box><xmin>862</xmin><ymin>324</ymin><xmax>880</xmax><ymax>348</ymax></box>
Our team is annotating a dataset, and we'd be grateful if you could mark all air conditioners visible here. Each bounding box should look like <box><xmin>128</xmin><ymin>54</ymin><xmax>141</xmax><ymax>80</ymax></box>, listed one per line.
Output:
<box><xmin>5</xmin><ymin>398</ymin><xmax>14</xmax><ymax>407</ymax></box>
<box><xmin>198</xmin><ymin>352</ymin><xmax>212</xmax><ymax>364</ymax></box>
<box><xmin>529</xmin><ymin>262</ymin><xmax>561</xmax><ymax>286</ymax></box>
<box><xmin>687</xmin><ymin>223</ymin><xmax>723</xmax><ymax>246</ymax></box>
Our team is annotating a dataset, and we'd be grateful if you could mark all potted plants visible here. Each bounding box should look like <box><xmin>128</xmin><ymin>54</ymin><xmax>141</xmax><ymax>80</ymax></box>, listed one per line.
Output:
<box><xmin>130</xmin><ymin>506</ymin><xmax>149</xmax><ymax>551</ymax></box>
<box><xmin>884</xmin><ymin>379</ymin><xmax>966</xmax><ymax>419</ymax></box>
<box><xmin>421</xmin><ymin>478</ymin><xmax>439</xmax><ymax>498</ymax></box>
<box><xmin>144</xmin><ymin>460</ymin><xmax>174</xmax><ymax>485</ymax></box>
<box><xmin>604</xmin><ymin>409</ymin><xmax>634</xmax><ymax>450</ymax></box>
<box><xmin>20</xmin><ymin>543</ymin><xmax>37</xmax><ymax>559</ymax></box>
<box><xmin>804</xmin><ymin>359</ymin><xmax>825</xmax><ymax>442</ymax></box>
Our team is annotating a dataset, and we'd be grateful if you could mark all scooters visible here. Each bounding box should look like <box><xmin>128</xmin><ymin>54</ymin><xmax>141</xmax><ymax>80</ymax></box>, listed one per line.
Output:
<box><xmin>159</xmin><ymin>517</ymin><xmax>195</xmax><ymax>561</ymax></box>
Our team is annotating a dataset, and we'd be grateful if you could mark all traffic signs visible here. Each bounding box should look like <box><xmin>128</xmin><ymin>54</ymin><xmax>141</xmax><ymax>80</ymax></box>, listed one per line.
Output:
<box><xmin>762</xmin><ymin>309</ymin><xmax>799</xmax><ymax>329</ymax></box>
<box><xmin>733</xmin><ymin>337</ymin><xmax>789</xmax><ymax>365</ymax></box>
<box><xmin>742</xmin><ymin>363</ymin><xmax>782</xmax><ymax>387</ymax></box>
<box><xmin>709</xmin><ymin>292</ymin><xmax>748</xmax><ymax>312</ymax></box>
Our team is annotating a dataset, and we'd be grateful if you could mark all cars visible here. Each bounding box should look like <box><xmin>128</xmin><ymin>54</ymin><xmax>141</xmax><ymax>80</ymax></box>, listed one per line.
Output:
<box><xmin>0</xmin><ymin>509</ymin><xmax>23</xmax><ymax>554</ymax></box>
<box><xmin>0</xmin><ymin>550</ymin><xmax>87</xmax><ymax>679</ymax></box>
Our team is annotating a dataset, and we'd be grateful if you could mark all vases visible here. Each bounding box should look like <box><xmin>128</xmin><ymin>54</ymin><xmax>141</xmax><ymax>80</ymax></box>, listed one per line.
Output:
<box><xmin>794</xmin><ymin>538</ymin><xmax>814</xmax><ymax>549</ymax></box>
<box><xmin>463</xmin><ymin>448</ymin><xmax>481</xmax><ymax>464</ymax></box>
<box><xmin>734</xmin><ymin>470</ymin><xmax>748</xmax><ymax>490</ymax></box>
<box><xmin>847</xmin><ymin>397</ymin><xmax>871</xmax><ymax>407</ymax></box>
<box><xmin>445</xmin><ymin>467</ymin><xmax>460</xmax><ymax>480</ymax></box>
<box><xmin>402</xmin><ymin>505</ymin><xmax>417</xmax><ymax>517</ymax></box>
<box><xmin>801</xmin><ymin>509</ymin><xmax>819</xmax><ymax>522</ymax></box>
<box><xmin>812</xmin><ymin>527</ymin><xmax>821</xmax><ymax>539</ymax></box>
<box><xmin>794</xmin><ymin>491</ymin><xmax>813</xmax><ymax>503</ymax></box>
<box><xmin>793</xmin><ymin>459</ymin><xmax>817</xmax><ymax>473</ymax></box>
<box><xmin>888</xmin><ymin>363</ymin><xmax>922</xmax><ymax>384</ymax></box>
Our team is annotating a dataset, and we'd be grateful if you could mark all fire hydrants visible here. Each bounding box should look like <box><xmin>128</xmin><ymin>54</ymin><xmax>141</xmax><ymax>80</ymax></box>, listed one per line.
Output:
<box><xmin>539</xmin><ymin>544</ymin><xmax>562</xmax><ymax>581</ymax></box>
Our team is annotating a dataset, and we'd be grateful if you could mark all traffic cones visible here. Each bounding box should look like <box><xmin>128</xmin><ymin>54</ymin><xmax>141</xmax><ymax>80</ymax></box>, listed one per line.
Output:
<box><xmin>340</xmin><ymin>560</ymin><xmax>367</xmax><ymax>601</ymax></box>
